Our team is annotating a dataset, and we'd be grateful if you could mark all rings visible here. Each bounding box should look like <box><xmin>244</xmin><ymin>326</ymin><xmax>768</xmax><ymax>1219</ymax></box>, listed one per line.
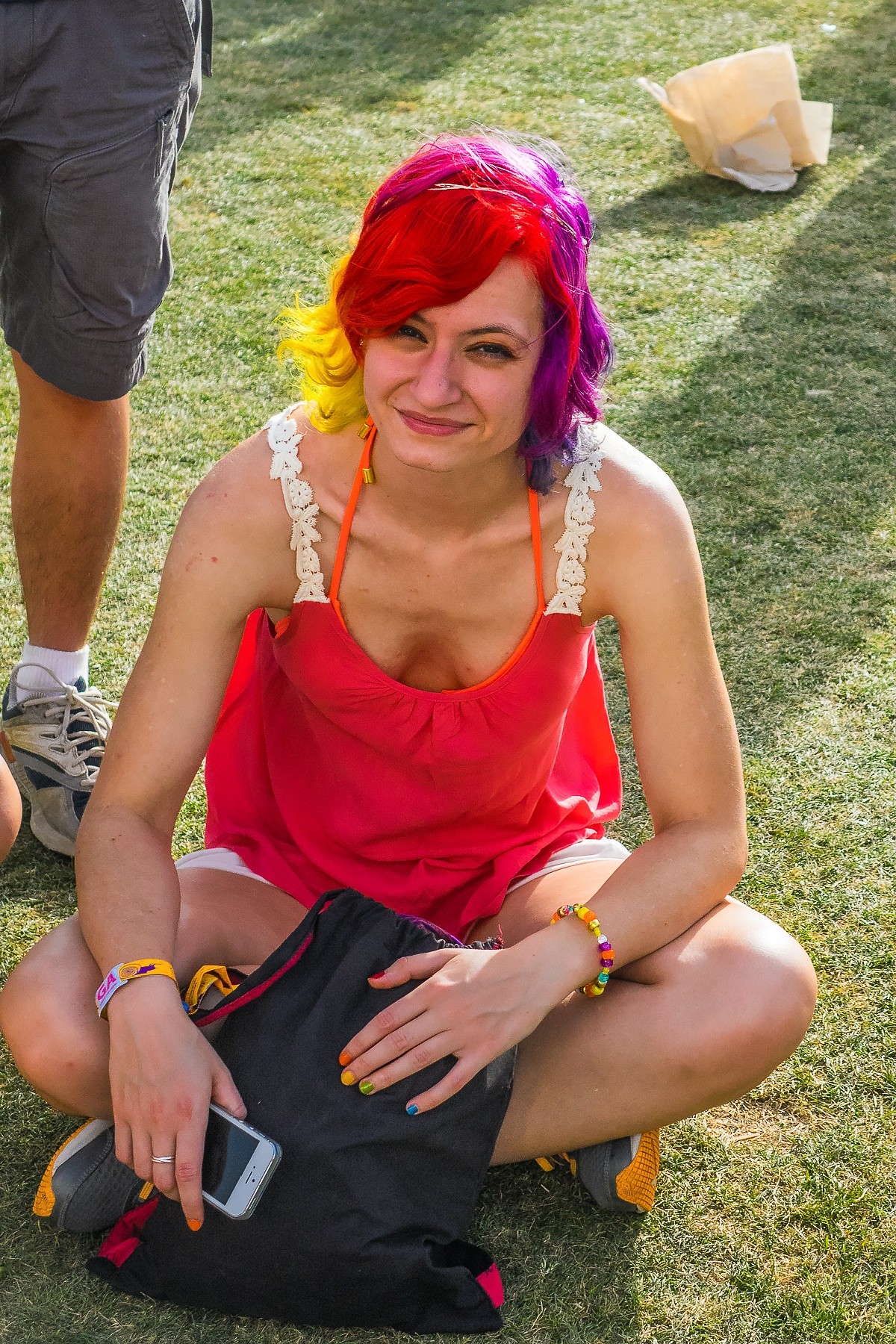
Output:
<box><xmin>150</xmin><ymin>1155</ymin><xmax>175</xmax><ymax>1164</ymax></box>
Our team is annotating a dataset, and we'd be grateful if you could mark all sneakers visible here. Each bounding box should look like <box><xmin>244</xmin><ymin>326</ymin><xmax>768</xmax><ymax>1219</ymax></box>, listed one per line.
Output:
<box><xmin>29</xmin><ymin>1119</ymin><xmax>164</xmax><ymax>1231</ymax></box>
<box><xmin>530</xmin><ymin>1126</ymin><xmax>662</xmax><ymax>1217</ymax></box>
<box><xmin>2</xmin><ymin>662</ymin><xmax>126</xmax><ymax>855</ymax></box>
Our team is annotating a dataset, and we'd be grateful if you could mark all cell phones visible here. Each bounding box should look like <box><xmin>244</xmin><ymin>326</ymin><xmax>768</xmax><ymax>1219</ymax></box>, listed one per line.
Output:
<box><xmin>202</xmin><ymin>1100</ymin><xmax>283</xmax><ymax>1222</ymax></box>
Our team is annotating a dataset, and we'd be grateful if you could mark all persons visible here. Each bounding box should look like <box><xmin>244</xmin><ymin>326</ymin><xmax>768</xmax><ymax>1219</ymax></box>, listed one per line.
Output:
<box><xmin>0</xmin><ymin>139</ymin><xmax>818</xmax><ymax>1242</ymax></box>
<box><xmin>0</xmin><ymin>0</ymin><xmax>218</xmax><ymax>859</ymax></box>
<box><xmin>2</xmin><ymin>753</ymin><xmax>24</xmax><ymax>918</ymax></box>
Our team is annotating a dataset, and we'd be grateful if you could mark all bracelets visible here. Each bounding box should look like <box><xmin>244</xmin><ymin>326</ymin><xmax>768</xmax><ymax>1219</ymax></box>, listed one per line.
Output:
<box><xmin>549</xmin><ymin>903</ymin><xmax>616</xmax><ymax>997</ymax></box>
<box><xmin>92</xmin><ymin>959</ymin><xmax>181</xmax><ymax>1023</ymax></box>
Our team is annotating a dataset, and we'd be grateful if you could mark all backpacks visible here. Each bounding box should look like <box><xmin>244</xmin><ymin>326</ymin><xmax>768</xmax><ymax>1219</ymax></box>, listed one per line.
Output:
<box><xmin>90</xmin><ymin>884</ymin><xmax>509</xmax><ymax>1338</ymax></box>
